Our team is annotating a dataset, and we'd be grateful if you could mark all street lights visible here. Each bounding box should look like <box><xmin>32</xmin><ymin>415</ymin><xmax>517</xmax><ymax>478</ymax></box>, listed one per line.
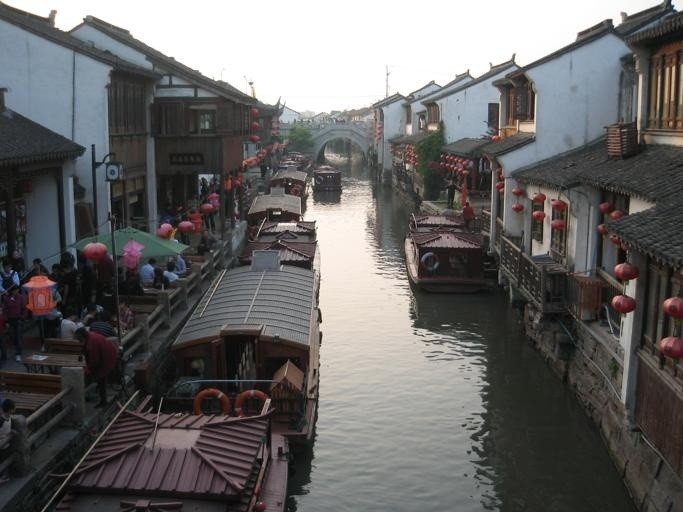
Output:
<box><xmin>91</xmin><ymin>143</ymin><xmax>125</xmax><ymax>237</ymax></box>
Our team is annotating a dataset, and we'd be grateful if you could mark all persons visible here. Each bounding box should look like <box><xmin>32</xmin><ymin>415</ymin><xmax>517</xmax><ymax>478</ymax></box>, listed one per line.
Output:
<box><xmin>461</xmin><ymin>201</ymin><xmax>476</xmax><ymax>232</ymax></box>
<box><xmin>439</xmin><ymin>180</ymin><xmax>463</xmax><ymax>209</ymax></box>
<box><xmin>1</xmin><ymin>400</ymin><xmax>18</xmax><ymax>483</ymax></box>
<box><xmin>1</xmin><ymin>255</ymin><xmax>187</xmax><ymax>409</ymax></box>
<box><xmin>159</xmin><ymin>171</ymin><xmax>250</xmax><ymax>256</ymax></box>
<box><xmin>259</xmin><ymin>151</ymin><xmax>283</xmax><ymax>178</ymax></box>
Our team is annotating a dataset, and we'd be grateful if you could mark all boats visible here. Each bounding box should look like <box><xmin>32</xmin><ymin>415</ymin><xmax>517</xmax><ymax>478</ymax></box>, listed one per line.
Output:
<box><xmin>247</xmin><ymin>188</ymin><xmax>303</xmax><ymax>224</ymax></box>
<box><xmin>311</xmin><ymin>165</ymin><xmax>343</xmax><ymax>191</ymax></box>
<box><xmin>161</xmin><ymin>248</ymin><xmax>321</xmax><ymax>446</ymax></box>
<box><xmin>40</xmin><ymin>395</ymin><xmax>289</xmax><ymax>512</ymax></box>
<box><xmin>267</xmin><ymin>150</ymin><xmax>312</xmax><ymax>203</ymax></box>
<box><xmin>404</xmin><ymin>209</ymin><xmax>493</xmax><ymax>293</ymax></box>
<box><xmin>238</xmin><ymin>217</ymin><xmax>321</xmax><ymax>296</ymax></box>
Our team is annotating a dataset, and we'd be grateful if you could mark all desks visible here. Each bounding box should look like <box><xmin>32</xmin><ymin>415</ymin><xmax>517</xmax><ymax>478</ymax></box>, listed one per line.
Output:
<box><xmin>0</xmin><ymin>390</ymin><xmax>53</xmax><ymax>432</ymax></box>
<box><xmin>19</xmin><ymin>351</ymin><xmax>87</xmax><ymax>374</ymax></box>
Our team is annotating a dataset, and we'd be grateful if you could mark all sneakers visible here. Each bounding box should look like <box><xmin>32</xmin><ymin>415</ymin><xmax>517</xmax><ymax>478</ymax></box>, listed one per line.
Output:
<box><xmin>95</xmin><ymin>403</ymin><xmax>107</xmax><ymax>408</ymax></box>
<box><xmin>14</xmin><ymin>354</ymin><xmax>21</xmax><ymax>361</ymax></box>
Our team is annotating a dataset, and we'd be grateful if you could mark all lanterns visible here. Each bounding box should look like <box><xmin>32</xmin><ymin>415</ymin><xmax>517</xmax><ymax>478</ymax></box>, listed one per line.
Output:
<box><xmin>593</xmin><ymin>201</ymin><xmax>630</xmax><ymax>251</ymax></box>
<box><xmin>84</xmin><ymin>242</ymin><xmax>107</xmax><ymax>260</ymax></box>
<box><xmin>531</xmin><ymin>210</ymin><xmax>545</xmax><ymax>221</ymax></box>
<box><xmin>511</xmin><ymin>203</ymin><xmax>524</xmax><ymax>213</ymax></box>
<box><xmin>612</xmin><ymin>264</ymin><xmax>639</xmax><ymax>281</ymax></box>
<box><xmin>549</xmin><ymin>219</ymin><xmax>564</xmax><ymax>229</ymax></box>
<box><xmin>549</xmin><ymin>199</ymin><xmax>568</xmax><ymax>212</ymax></box>
<box><xmin>511</xmin><ymin>188</ymin><xmax>524</xmax><ymax>196</ymax></box>
<box><xmin>250</xmin><ymin>109</ymin><xmax>283</xmax><ymax>166</ymax></box>
<box><xmin>661</xmin><ymin>296</ymin><xmax>683</xmax><ymax>317</ymax></box>
<box><xmin>609</xmin><ymin>294</ymin><xmax>635</xmax><ymax>313</ymax></box>
<box><xmin>531</xmin><ymin>192</ymin><xmax>546</xmax><ymax>203</ymax></box>
<box><xmin>659</xmin><ymin>336</ymin><xmax>682</xmax><ymax>357</ymax></box>
<box><xmin>376</xmin><ymin>123</ymin><xmax>505</xmax><ymax>195</ymax></box>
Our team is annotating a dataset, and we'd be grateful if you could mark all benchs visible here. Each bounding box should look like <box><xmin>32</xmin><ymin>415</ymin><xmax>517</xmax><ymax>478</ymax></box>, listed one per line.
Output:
<box><xmin>41</xmin><ymin>254</ymin><xmax>204</xmax><ymax>353</ymax></box>
<box><xmin>0</xmin><ymin>370</ymin><xmax>61</xmax><ymax>393</ymax></box>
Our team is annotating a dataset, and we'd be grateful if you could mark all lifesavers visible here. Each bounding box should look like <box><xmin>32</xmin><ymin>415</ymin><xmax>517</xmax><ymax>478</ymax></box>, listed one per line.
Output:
<box><xmin>420</xmin><ymin>252</ymin><xmax>439</xmax><ymax>272</ymax></box>
<box><xmin>235</xmin><ymin>389</ymin><xmax>268</xmax><ymax>418</ymax></box>
<box><xmin>316</xmin><ymin>176</ymin><xmax>323</xmax><ymax>184</ymax></box>
<box><xmin>291</xmin><ymin>184</ymin><xmax>303</xmax><ymax>197</ymax></box>
<box><xmin>193</xmin><ymin>389</ymin><xmax>231</xmax><ymax>416</ymax></box>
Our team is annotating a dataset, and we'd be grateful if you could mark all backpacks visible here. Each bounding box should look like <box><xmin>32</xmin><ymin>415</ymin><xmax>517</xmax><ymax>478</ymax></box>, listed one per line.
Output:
<box><xmin>1</xmin><ymin>271</ymin><xmax>16</xmax><ymax>291</ymax></box>
